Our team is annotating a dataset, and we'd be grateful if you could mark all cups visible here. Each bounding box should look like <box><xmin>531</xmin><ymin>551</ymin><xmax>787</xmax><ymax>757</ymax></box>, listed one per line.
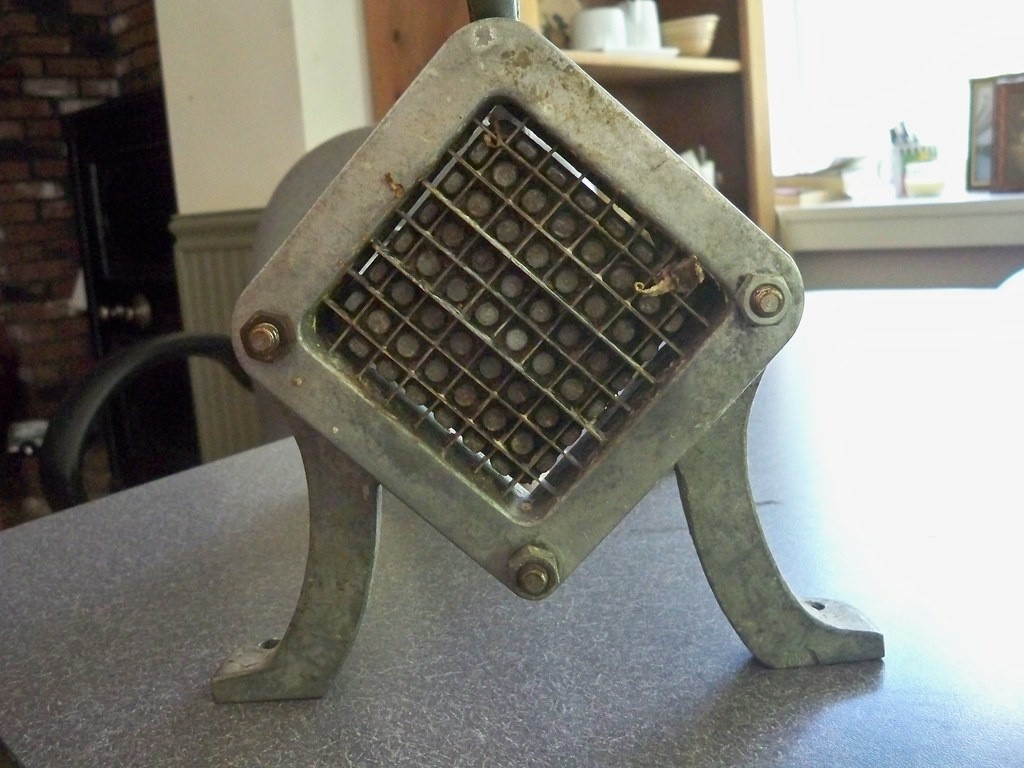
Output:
<box><xmin>571</xmin><ymin>0</ymin><xmax>664</xmax><ymax>48</ymax></box>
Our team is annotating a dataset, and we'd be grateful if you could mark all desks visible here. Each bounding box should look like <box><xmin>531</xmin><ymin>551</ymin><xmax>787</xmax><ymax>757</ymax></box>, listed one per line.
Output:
<box><xmin>0</xmin><ymin>289</ymin><xmax>1024</xmax><ymax>767</ymax></box>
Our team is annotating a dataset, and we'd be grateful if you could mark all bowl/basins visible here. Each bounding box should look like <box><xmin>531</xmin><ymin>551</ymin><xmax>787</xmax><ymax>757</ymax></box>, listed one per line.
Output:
<box><xmin>660</xmin><ymin>15</ymin><xmax>720</xmax><ymax>56</ymax></box>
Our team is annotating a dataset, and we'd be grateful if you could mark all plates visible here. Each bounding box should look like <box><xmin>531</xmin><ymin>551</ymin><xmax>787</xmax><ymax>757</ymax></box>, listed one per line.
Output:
<box><xmin>582</xmin><ymin>47</ymin><xmax>681</xmax><ymax>56</ymax></box>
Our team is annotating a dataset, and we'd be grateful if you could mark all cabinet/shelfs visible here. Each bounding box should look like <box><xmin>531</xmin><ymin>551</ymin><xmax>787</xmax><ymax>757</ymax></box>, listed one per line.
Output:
<box><xmin>362</xmin><ymin>0</ymin><xmax>775</xmax><ymax>255</ymax></box>
<box><xmin>58</xmin><ymin>88</ymin><xmax>202</xmax><ymax>485</ymax></box>
